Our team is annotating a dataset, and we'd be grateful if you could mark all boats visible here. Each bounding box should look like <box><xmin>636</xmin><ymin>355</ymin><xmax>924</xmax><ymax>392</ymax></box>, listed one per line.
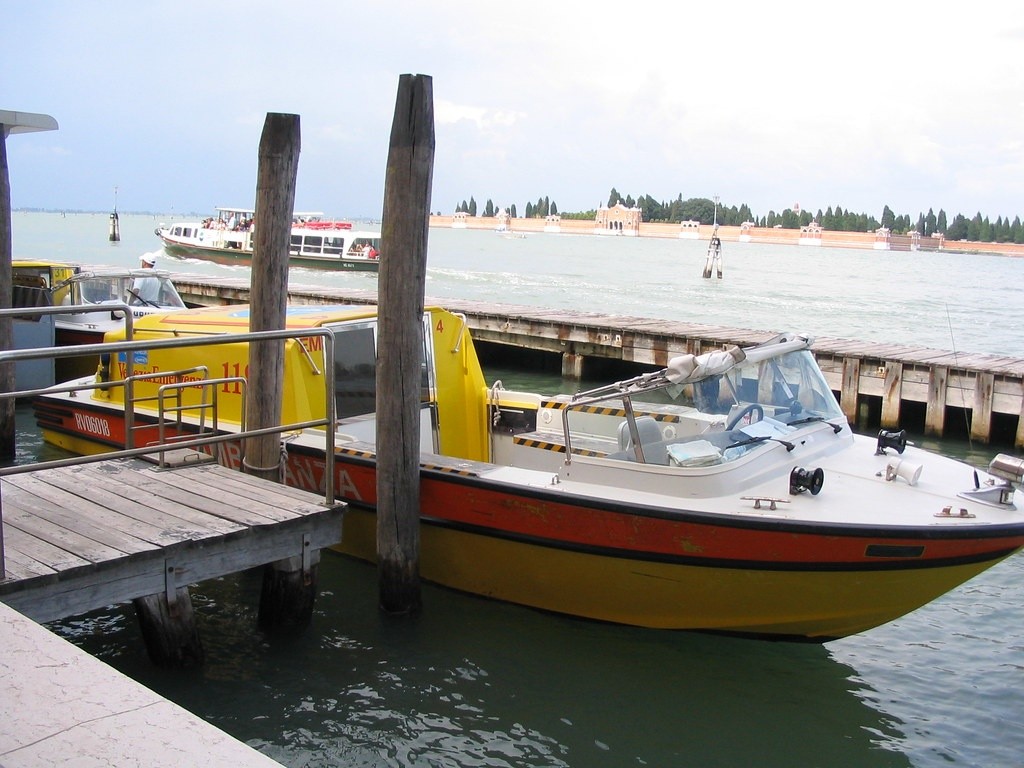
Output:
<box><xmin>154</xmin><ymin>208</ymin><xmax>382</xmax><ymax>274</ymax></box>
<box><xmin>34</xmin><ymin>303</ymin><xmax>1022</xmax><ymax>644</ymax></box>
<box><xmin>11</xmin><ymin>257</ymin><xmax>191</xmax><ymax>387</ymax></box>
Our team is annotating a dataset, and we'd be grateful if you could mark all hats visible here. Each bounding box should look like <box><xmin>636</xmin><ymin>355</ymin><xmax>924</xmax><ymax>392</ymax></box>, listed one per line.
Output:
<box><xmin>138</xmin><ymin>252</ymin><xmax>155</xmax><ymax>265</ymax></box>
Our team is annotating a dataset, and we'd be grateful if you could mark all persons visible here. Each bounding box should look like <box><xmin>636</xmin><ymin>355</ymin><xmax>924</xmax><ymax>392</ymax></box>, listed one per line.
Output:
<box><xmin>128</xmin><ymin>252</ymin><xmax>161</xmax><ymax>306</ymax></box>
<box><xmin>200</xmin><ymin>211</ymin><xmax>382</xmax><ymax>260</ymax></box>
